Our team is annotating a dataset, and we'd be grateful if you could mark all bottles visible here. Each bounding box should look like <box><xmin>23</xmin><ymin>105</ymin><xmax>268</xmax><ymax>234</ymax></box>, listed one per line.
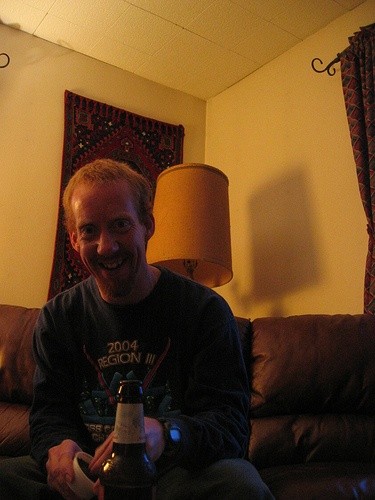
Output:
<box><xmin>97</xmin><ymin>381</ymin><xmax>157</xmax><ymax>500</ymax></box>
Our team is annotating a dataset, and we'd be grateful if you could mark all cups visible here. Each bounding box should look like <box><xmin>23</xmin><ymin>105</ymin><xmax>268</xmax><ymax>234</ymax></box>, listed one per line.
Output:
<box><xmin>67</xmin><ymin>452</ymin><xmax>97</xmax><ymax>498</ymax></box>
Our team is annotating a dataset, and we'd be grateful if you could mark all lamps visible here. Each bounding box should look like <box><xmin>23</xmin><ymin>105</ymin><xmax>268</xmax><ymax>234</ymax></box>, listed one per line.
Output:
<box><xmin>146</xmin><ymin>162</ymin><xmax>233</xmax><ymax>288</ymax></box>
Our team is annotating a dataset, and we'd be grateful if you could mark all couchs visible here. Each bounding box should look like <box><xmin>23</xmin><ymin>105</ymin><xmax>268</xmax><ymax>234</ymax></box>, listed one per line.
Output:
<box><xmin>1</xmin><ymin>304</ymin><xmax>375</xmax><ymax>500</ymax></box>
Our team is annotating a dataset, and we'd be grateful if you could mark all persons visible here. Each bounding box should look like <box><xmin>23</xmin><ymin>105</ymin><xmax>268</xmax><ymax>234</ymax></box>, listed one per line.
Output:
<box><xmin>0</xmin><ymin>159</ymin><xmax>272</xmax><ymax>500</ymax></box>
